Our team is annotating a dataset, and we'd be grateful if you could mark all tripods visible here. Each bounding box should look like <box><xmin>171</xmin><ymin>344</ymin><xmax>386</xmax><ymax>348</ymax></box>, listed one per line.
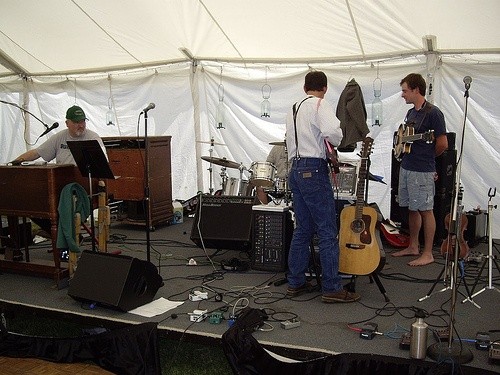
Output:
<box><xmin>418</xmin><ymin>185</ymin><xmax>500</xmax><ymax>310</ymax></box>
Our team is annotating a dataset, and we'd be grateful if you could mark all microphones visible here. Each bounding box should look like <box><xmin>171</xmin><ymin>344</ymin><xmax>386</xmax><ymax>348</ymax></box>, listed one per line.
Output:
<box><xmin>141</xmin><ymin>103</ymin><xmax>155</xmax><ymax>114</ymax></box>
<box><xmin>40</xmin><ymin>122</ymin><xmax>59</xmax><ymax>137</ymax></box>
<box><xmin>463</xmin><ymin>76</ymin><xmax>472</xmax><ymax>89</ymax></box>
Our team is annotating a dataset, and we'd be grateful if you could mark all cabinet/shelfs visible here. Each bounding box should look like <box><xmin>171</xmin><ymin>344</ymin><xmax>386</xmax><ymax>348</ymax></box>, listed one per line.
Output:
<box><xmin>101</xmin><ymin>134</ymin><xmax>173</xmax><ymax>231</ymax></box>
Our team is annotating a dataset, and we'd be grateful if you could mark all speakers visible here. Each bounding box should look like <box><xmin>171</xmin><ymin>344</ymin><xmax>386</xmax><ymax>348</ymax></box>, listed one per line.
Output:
<box><xmin>67</xmin><ymin>249</ymin><xmax>164</xmax><ymax>313</ymax></box>
<box><xmin>190</xmin><ymin>194</ymin><xmax>255</xmax><ymax>252</ymax></box>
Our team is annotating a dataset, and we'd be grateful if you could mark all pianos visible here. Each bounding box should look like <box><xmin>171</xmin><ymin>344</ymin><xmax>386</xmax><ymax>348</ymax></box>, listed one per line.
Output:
<box><xmin>0</xmin><ymin>164</ymin><xmax>122</xmax><ymax>290</ymax></box>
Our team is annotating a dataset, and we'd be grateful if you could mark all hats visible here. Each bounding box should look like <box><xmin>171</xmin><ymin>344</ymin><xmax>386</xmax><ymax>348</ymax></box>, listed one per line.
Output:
<box><xmin>65</xmin><ymin>105</ymin><xmax>89</xmax><ymax>122</ymax></box>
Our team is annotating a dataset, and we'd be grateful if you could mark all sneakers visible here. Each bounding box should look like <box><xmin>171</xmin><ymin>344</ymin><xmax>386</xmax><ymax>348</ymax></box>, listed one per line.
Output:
<box><xmin>286</xmin><ymin>282</ymin><xmax>313</xmax><ymax>295</ymax></box>
<box><xmin>322</xmin><ymin>288</ymin><xmax>361</xmax><ymax>303</ymax></box>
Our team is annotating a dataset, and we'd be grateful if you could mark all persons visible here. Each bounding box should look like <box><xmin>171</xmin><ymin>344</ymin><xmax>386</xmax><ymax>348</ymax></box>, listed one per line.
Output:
<box><xmin>267</xmin><ymin>132</ymin><xmax>289</xmax><ymax>181</ymax></box>
<box><xmin>8</xmin><ymin>105</ymin><xmax>110</xmax><ymax>248</ymax></box>
<box><xmin>284</xmin><ymin>71</ymin><xmax>361</xmax><ymax>303</ymax></box>
<box><xmin>390</xmin><ymin>73</ymin><xmax>449</xmax><ymax>266</ymax></box>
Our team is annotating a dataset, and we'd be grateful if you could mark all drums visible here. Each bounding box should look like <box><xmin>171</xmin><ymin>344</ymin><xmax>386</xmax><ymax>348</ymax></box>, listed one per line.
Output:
<box><xmin>220</xmin><ymin>178</ymin><xmax>254</xmax><ymax>197</ymax></box>
<box><xmin>249</xmin><ymin>161</ymin><xmax>278</xmax><ymax>186</ymax></box>
<box><xmin>262</xmin><ymin>177</ymin><xmax>292</xmax><ymax>194</ymax></box>
<box><xmin>266</xmin><ymin>195</ymin><xmax>297</xmax><ymax>229</ymax></box>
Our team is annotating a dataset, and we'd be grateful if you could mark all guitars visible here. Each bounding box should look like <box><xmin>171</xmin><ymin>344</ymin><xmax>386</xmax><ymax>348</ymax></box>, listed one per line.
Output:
<box><xmin>394</xmin><ymin>123</ymin><xmax>435</xmax><ymax>159</ymax></box>
<box><xmin>337</xmin><ymin>136</ymin><xmax>387</xmax><ymax>275</ymax></box>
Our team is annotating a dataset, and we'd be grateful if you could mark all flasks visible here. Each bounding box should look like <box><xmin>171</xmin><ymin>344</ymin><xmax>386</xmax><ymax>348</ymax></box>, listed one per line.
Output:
<box><xmin>410</xmin><ymin>310</ymin><xmax>428</xmax><ymax>357</ymax></box>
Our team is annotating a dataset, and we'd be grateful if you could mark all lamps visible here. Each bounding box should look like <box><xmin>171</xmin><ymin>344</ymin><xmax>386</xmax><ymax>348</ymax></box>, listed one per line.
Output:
<box><xmin>214</xmin><ymin>66</ymin><xmax>227</xmax><ymax>129</ymax></box>
<box><xmin>424</xmin><ymin>71</ymin><xmax>434</xmax><ymax>104</ymax></box>
<box><xmin>372</xmin><ymin>62</ymin><xmax>382</xmax><ymax>127</ymax></box>
<box><xmin>260</xmin><ymin>64</ymin><xmax>272</xmax><ymax>117</ymax></box>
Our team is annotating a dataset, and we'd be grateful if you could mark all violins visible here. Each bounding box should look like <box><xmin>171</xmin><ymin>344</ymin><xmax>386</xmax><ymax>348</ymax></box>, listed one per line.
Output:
<box><xmin>440</xmin><ymin>191</ymin><xmax>469</xmax><ymax>261</ymax></box>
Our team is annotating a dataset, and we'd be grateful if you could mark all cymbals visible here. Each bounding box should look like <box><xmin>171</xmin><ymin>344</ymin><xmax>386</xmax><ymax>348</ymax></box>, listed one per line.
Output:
<box><xmin>201</xmin><ymin>156</ymin><xmax>246</xmax><ymax>170</ymax></box>
<box><xmin>195</xmin><ymin>140</ymin><xmax>228</xmax><ymax>146</ymax></box>
<box><xmin>268</xmin><ymin>142</ymin><xmax>287</xmax><ymax>146</ymax></box>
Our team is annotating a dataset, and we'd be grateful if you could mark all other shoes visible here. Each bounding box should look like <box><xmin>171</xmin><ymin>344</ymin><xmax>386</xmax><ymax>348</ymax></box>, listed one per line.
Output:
<box><xmin>78</xmin><ymin>234</ymin><xmax>84</xmax><ymax>246</ymax></box>
